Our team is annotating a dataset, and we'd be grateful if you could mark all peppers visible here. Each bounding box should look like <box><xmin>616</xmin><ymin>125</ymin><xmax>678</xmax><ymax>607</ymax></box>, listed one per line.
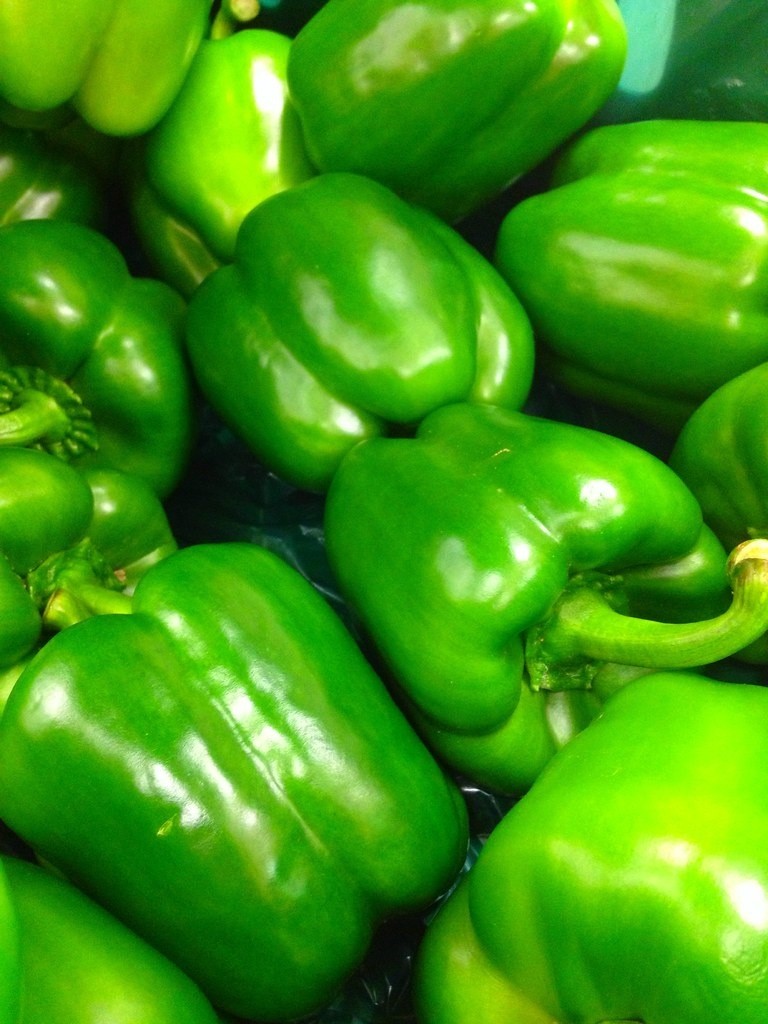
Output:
<box><xmin>0</xmin><ymin>0</ymin><xmax>768</xmax><ymax>1024</ymax></box>
